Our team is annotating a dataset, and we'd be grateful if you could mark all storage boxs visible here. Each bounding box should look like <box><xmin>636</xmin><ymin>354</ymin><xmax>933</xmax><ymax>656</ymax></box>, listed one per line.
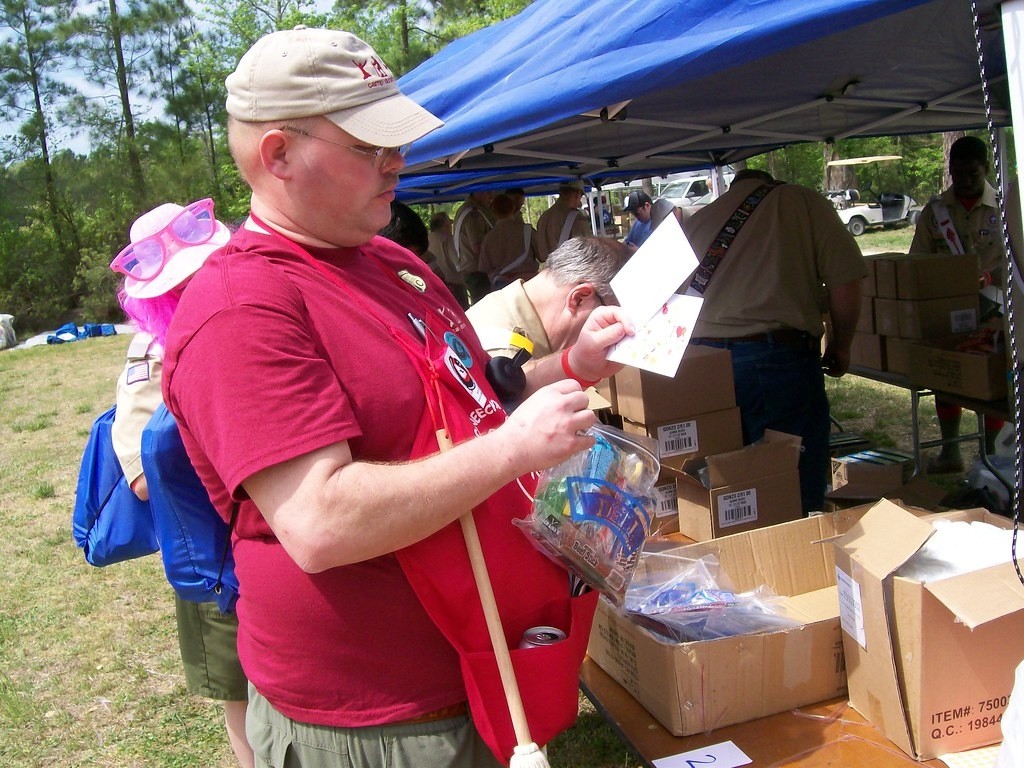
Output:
<box><xmin>578</xmin><ymin>250</ymin><xmax>1024</xmax><ymax>760</ymax></box>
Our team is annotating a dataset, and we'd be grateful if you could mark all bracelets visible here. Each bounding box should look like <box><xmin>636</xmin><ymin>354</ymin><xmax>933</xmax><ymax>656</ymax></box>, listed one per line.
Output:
<box><xmin>561</xmin><ymin>345</ymin><xmax>601</xmax><ymax>387</ymax></box>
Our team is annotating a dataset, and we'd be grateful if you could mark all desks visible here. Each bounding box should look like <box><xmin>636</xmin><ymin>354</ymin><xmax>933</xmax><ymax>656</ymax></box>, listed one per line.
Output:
<box><xmin>578</xmin><ymin>656</ymin><xmax>948</xmax><ymax>768</ymax></box>
<box><xmin>819</xmin><ymin>354</ymin><xmax>1024</xmax><ymax>511</ymax></box>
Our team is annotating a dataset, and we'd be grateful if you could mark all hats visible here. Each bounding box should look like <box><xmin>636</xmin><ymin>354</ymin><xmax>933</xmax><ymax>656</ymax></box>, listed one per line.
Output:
<box><xmin>225</xmin><ymin>25</ymin><xmax>445</xmax><ymax>148</ymax></box>
<box><xmin>559</xmin><ymin>179</ymin><xmax>589</xmax><ymax>199</ymax></box>
<box><xmin>123</xmin><ymin>202</ymin><xmax>230</xmax><ymax>299</ymax></box>
<box><xmin>624</xmin><ymin>191</ymin><xmax>653</xmax><ymax>212</ymax></box>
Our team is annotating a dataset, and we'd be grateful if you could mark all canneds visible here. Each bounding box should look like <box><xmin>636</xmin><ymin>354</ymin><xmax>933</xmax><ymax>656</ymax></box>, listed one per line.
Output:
<box><xmin>519</xmin><ymin>626</ymin><xmax>567</xmax><ymax>649</ymax></box>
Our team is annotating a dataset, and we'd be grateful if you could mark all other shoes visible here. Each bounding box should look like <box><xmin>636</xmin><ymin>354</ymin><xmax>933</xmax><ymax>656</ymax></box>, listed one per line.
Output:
<box><xmin>923</xmin><ymin>450</ymin><xmax>965</xmax><ymax>474</ymax></box>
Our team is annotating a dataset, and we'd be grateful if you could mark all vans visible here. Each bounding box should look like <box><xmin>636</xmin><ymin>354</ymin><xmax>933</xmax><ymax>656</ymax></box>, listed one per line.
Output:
<box><xmin>548</xmin><ymin>162</ymin><xmax>735</xmax><ymax>242</ymax></box>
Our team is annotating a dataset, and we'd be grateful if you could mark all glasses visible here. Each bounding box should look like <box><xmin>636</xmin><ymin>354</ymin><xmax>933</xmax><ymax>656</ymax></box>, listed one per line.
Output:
<box><xmin>110</xmin><ymin>197</ymin><xmax>217</xmax><ymax>282</ymax></box>
<box><xmin>631</xmin><ymin>207</ymin><xmax>643</xmax><ymax>218</ymax></box>
<box><xmin>278</xmin><ymin>125</ymin><xmax>412</xmax><ymax>168</ymax></box>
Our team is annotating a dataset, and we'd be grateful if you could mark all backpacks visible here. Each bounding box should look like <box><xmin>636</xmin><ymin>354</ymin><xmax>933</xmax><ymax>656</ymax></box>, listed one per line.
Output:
<box><xmin>73</xmin><ymin>409</ymin><xmax>166</xmax><ymax>567</ymax></box>
<box><xmin>141</xmin><ymin>399</ymin><xmax>243</xmax><ymax>615</ymax></box>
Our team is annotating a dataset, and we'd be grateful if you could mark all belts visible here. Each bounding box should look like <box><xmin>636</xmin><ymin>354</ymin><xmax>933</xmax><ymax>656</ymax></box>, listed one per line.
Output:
<box><xmin>701</xmin><ymin>328</ymin><xmax>813</xmax><ymax>343</ymax></box>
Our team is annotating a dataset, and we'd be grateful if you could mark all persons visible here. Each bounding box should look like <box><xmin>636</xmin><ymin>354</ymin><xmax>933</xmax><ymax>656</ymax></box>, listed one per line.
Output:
<box><xmin>111</xmin><ymin>201</ymin><xmax>256</xmax><ymax>768</ymax></box>
<box><xmin>620</xmin><ymin>191</ymin><xmax>653</xmax><ymax>253</ymax></box>
<box><xmin>162</xmin><ymin>16</ymin><xmax>634</xmax><ymax>767</ymax></box>
<box><xmin>453</xmin><ymin>188</ymin><xmax>538</xmax><ymax>305</ymax></box>
<box><xmin>649</xmin><ymin>198</ymin><xmax>707</xmax><ymax>230</ymax></box>
<box><xmin>674</xmin><ymin>167</ymin><xmax>870</xmax><ymax>517</ymax></box>
<box><xmin>427</xmin><ymin>212</ymin><xmax>469</xmax><ymax>312</ymax></box>
<box><xmin>378</xmin><ymin>200</ymin><xmax>429</xmax><ymax>258</ymax></box>
<box><xmin>462</xmin><ymin>236</ymin><xmax>636</xmax><ymax>362</ymax></box>
<box><xmin>536</xmin><ymin>180</ymin><xmax>594</xmax><ymax>263</ymax></box>
<box><xmin>909</xmin><ymin>136</ymin><xmax>1008</xmax><ymax>475</ymax></box>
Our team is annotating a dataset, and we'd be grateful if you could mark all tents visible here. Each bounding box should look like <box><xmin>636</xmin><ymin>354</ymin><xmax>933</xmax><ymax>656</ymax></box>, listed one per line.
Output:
<box><xmin>385</xmin><ymin>0</ymin><xmax>1008</xmax><ymax>240</ymax></box>
